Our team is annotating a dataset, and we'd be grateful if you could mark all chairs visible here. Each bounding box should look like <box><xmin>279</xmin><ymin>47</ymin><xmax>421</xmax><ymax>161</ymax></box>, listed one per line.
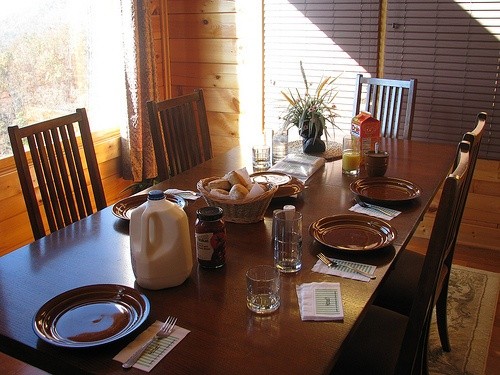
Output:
<box><xmin>146</xmin><ymin>88</ymin><xmax>213</xmax><ymax>182</ymax></box>
<box><xmin>329</xmin><ymin>74</ymin><xmax>487</xmax><ymax>375</ymax></box>
<box><xmin>8</xmin><ymin>107</ymin><xmax>107</xmax><ymax>240</ymax></box>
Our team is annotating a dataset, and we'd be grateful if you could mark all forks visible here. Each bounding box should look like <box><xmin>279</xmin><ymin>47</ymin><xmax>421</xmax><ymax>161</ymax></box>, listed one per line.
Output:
<box><xmin>177</xmin><ymin>190</ymin><xmax>200</xmax><ymax>196</ymax></box>
<box><xmin>354</xmin><ymin>197</ymin><xmax>392</xmax><ymax>217</ymax></box>
<box><xmin>316</xmin><ymin>252</ymin><xmax>376</xmax><ymax>279</ymax></box>
<box><xmin>122</xmin><ymin>315</ymin><xmax>177</xmax><ymax>369</ymax></box>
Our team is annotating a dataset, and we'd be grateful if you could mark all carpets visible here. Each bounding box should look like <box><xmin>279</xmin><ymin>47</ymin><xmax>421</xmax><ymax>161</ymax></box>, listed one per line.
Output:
<box><xmin>418</xmin><ymin>264</ymin><xmax>500</xmax><ymax>374</ymax></box>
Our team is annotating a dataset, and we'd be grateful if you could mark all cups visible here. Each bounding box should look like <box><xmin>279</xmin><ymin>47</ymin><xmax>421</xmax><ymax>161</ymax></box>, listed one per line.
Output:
<box><xmin>341</xmin><ymin>135</ymin><xmax>361</xmax><ymax>177</ymax></box>
<box><xmin>245</xmin><ymin>264</ymin><xmax>281</xmax><ymax>315</ymax></box>
<box><xmin>271</xmin><ymin>128</ymin><xmax>289</xmax><ymax>165</ymax></box>
<box><xmin>366</xmin><ymin>150</ymin><xmax>390</xmax><ymax>177</ymax></box>
<box><xmin>272</xmin><ymin>209</ymin><xmax>303</xmax><ymax>274</ymax></box>
<box><xmin>253</xmin><ymin>144</ymin><xmax>271</xmax><ymax>173</ymax></box>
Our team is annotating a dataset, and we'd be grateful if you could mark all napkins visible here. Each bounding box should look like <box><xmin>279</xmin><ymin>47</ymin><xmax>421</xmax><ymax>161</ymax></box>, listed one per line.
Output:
<box><xmin>311</xmin><ymin>256</ymin><xmax>377</xmax><ymax>282</ymax></box>
<box><xmin>112</xmin><ymin>319</ymin><xmax>191</xmax><ymax>373</ymax></box>
<box><xmin>163</xmin><ymin>188</ymin><xmax>202</xmax><ymax>200</ymax></box>
<box><xmin>348</xmin><ymin>201</ymin><xmax>402</xmax><ymax>221</ymax></box>
<box><xmin>295</xmin><ymin>282</ymin><xmax>344</xmax><ymax>321</ymax></box>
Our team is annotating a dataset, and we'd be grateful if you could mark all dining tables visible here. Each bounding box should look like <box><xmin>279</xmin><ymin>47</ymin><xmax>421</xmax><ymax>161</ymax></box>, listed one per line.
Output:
<box><xmin>0</xmin><ymin>140</ymin><xmax>461</xmax><ymax>375</ymax></box>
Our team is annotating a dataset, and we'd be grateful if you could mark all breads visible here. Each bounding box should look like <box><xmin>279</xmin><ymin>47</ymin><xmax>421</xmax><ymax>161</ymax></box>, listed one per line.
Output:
<box><xmin>208</xmin><ymin>170</ymin><xmax>267</xmax><ymax>199</ymax></box>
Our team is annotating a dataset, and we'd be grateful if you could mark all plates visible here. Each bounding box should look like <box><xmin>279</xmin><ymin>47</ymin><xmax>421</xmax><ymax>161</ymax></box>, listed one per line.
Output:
<box><xmin>308</xmin><ymin>213</ymin><xmax>397</xmax><ymax>251</ymax></box>
<box><xmin>111</xmin><ymin>193</ymin><xmax>186</xmax><ymax>221</ymax></box>
<box><xmin>349</xmin><ymin>176</ymin><xmax>424</xmax><ymax>203</ymax></box>
<box><xmin>249</xmin><ymin>170</ymin><xmax>292</xmax><ymax>186</ymax></box>
<box><xmin>273</xmin><ymin>176</ymin><xmax>305</xmax><ymax>199</ymax></box>
<box><xmin>32</xmin><ymin>283</ymin><xmax>152</xmax><ymax>348</ymax></box>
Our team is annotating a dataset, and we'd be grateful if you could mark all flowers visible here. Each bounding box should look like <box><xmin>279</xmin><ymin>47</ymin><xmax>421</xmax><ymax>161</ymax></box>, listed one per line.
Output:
<box><xmin>279</xmin><ymin>60</ymin><xmax>341</xmax><ymax>145</ymax></box>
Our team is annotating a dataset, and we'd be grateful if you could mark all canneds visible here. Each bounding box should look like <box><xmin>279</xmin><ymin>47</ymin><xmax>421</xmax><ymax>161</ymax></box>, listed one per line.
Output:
<box><xmin>195</xmin><ymin>206</ymin><xmax>227</xmax><ymax>268</ymax></box>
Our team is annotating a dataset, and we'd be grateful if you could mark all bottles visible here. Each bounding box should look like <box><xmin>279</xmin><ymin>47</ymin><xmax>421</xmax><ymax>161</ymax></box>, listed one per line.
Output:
<box><xmin>129</xmin><ymin>189</ymin><xmax>193</xmax><ymax>290</ymax></box>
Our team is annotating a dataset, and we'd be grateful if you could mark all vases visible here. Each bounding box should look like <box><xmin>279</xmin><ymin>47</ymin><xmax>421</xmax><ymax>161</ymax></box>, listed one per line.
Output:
<box><xmin>298</xmin><ymin>117</ymin><xmax>325</xmax><ymax>152</ymax></box>
